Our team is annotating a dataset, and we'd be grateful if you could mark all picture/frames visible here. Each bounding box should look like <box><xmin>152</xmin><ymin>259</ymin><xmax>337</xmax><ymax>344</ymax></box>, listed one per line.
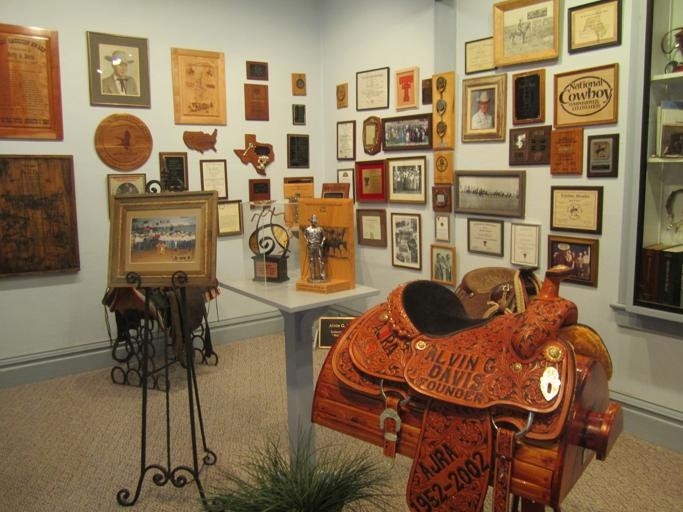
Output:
<box><xmin>493</xmin><ymin>0</ymin><xmax>559</xmax><ymax>66</ymax></box>
<box><xmin>284</xmin><ymin>177</ymin><xmax>313</xmax><ymax>183</ymax></box>
<box><xmin>356</xmin><ymin>208</ymin><xmax>386</xmax><ymax>246</ymax></box>
<box><xmin>390</xmin><ymin>212</ymin><xmax>422</xmax><ymax>270</ymax></box>
<box><xmin>200</xmin><ymin>159</ymin><xmax>228</xmax><ymax>199</ymax></box>
<box><xmin>355</xmin><ymin>159</ymin><xmax>386</xmax><ymax>203</ymax></box>
<box><xmin>430</xmin><ymin>244</ymin><xmax>457</xmax><ymax>287</ymax></box>
<box><xmin>551</xmin><ymin>126</ymin><xmax>583</xmax><ymax>175</ymax></box>
<box><xmin>362</xmin><ymin>116</ymin><xmax>381</xmax><ymax>155</ymax></box>
<box><xmin>336</xmin><ymin>83</ymin><xmax>348</xmax><ymax>108</ymax></box>
<box><xmin>171</xmin><ymin>48</ymin><xmax>226</xmax><ymax>126</ymax></box>
<box><xmin>433</xmin><ymin>151</ymin><xmax>453</xmax><ymax>185</ymax></box>
<box><xmin>567</xmin><ymin>0</ymin><xmax>622</xmax><ymax>53</ymax></box>
<box><xmin>106</xmin><ymin>189</ymin><xmax>217</xmax><ymax>285</ymax></box>
<box><xmin>217</xmin><ymin>199</ymin><xmax>244</xmax><ymax>236</ymax></box>
<box><xmin>549</xmin><ymin>185</ymin><xmax>604</xmax><ymax>235</ymax></box>
<box><xmin>356</xmin><ymin>67</ymin><xmax>390</xmax><ymax>111</ymax></box>
<box><xmin>284</xmin><ymin>183</ymin><xmax>314</xmax><ymax>228</ymax></box>
<box><xmin>0</xmin><ymin>154</ymin><xmax>81</xmax><ymax>277</ymax></box>
<box><xmin>1</xmin><ymin>23</ymin><xmax>64</xmax><ymax>141</ymax></box>
<box><xmin>432</xmin><ymin>71</ymin><xmax>455</xmax><ymax>150</ymax></box>
<box><xmin>291</xmin><ymin>73</ymin><xmax>306</xmax><ymax>96</ymax></box>
<box><xmin>432</xmin><ymin>185</ymin><xmax>452</xmax><ymax>212</ymax></box>
<box><xmin>381</xmin><ymin>113</ymin><xmax>432</xmax><ymax>151</ymax></box>
<box><xmin>107</xmin><ymin>173</ymin><xmax>147</xmax><ymax>219</ymax></box>
<box><xmin>87</xmin><ymin>31</ymin><xmax>151</xmax><ymax>109</ymax></box>
<box><xmin>394</xmin><ymin>66</ymin><xmax>419</xmax><ymax>109</ymax></box>
<box><xmin>243</xmin><ymin>84</ymin><xmax>270</xmax><ymax>121</ymax></box>
<box><xmin>336</xmin><ymin>120</ymin><xmax>356</xmax><ymax>160</ymax></box>
<box><xmin>434</xmin><ymin>215</ymin><xmax>450</xmax><ymax>243</ymax></box>
<box><xmin>512</xmin><ymin>69</ymin><xmax>545</xmax><ymax>124</ymax></box>
<box><xmin>337</xmin><ymin>168</ymin><xmax>354</xmax><ymax>203</ymax></box>
<box><xmin>464</xmin><ymin>35</ymin><xmax>498</xmax><ymax>74</ymax></box>
<box><xmin>461</xmin><ymin>72</ymin><xmax>508</xmax><ymax>143</ymax></box>
<box><xmin>293</xmin><ymin>104</ymin><xmax>306</xmax><ymax>125</ymax></box>
<box><xmin>467</xmin><ymin>217</ymin><xmax>504</xmax><ymax>257</ymax></box>
<box><xmin>287</xmin><ymin>133</ymin><xmax>310</xmax><ymax>169</ymax></box>
<box><xmin>454</xmin><ymin>170</ymin><xmax>526</xmax><ymax>219</ymax></box>
<box><xmin>386</xmin><ymin>154</ymin><xmax>426</xmax><ymax>203</ymax></box>
<box><xmin>548</xmin><ymin>235</ymin><xmax>598</xmax><ymax>287</ymax></box>
<box><xmin>322</xmin><ymin>183</ymin><xmax>350</xmax><ymax>199</ymax></box>
<box><xmin>509</xmin><ymin>123</ymin><xmax>552</xmax><ymax>165</ymax></box>
<box><xmin>553</xmin><ymin>62</ymin><xmax>619</xmax><ymax>128</ymax></box>
<box><xmin>248</xmin><ymin>179</ymin><xmax>271</xmax><ymax>209</ymax></box>
<box><xmin>510</xmin><ymin>223</ymin><xmax>540</xmax><ymax>266</ymax></box>
<box><xmin>246</xmin><ymin>60</ymin><xmax>269</xmax><ymax>81</ymax></box>
<box><xmin>586</xmin><ymin>133</ymin><xmax>619</xmax><ymax>177</ymax></box>
<box><xmin>159</xmin><ymin>152</ymin><xmax>189</xmax><ymax>192</ymax></box>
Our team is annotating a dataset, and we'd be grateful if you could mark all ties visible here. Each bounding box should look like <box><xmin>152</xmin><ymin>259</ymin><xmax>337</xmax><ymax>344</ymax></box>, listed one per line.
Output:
<box><xmin>119</xmin><ymin>79</ymin><xmax>126</xmax><ymax>92</ymax></box>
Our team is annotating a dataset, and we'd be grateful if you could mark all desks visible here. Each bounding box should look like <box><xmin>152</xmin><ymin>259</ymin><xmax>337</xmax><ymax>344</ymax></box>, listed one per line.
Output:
<box><xmin>217</xmin><ymin>279</ymin><xmax>381</xmax><ymax>475</ymax></box>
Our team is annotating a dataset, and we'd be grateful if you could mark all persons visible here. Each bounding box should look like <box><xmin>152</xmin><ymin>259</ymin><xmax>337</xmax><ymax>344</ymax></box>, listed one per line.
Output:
<box><xmin>392</xmin><ymin>168</ymin><xmax>420</xmax><ymax>192</ymax></box>
<box><xmin>517</xmin><ymin>18</ymin><xmax>524</xmax><ymax>34</ymax></box>
<box><xmin>102</xmin><ymin>52</ymin><xmax>138</xmax><ymax>96</ymax></box>
<box><xmin>434</xmin><ymin>254</ymin><xmax>451</xmax><ymax>282</ymax></box>
<box><xmin>553</xmin><ymin>250</ymin><xmax>590</xmax><ymax>278</ymax></box>
<box><xmin>385</xmin><ymin>125</ymin><xmax>426</xmax><ymax>144</ymax></box>
<box><xmin>470</xmin><ymin>92</ymin><xmax>494</xmax><ymax>129</ymax></box>
<box><xmin>303</xmin><ymin>214</ymin><xmax>327</xmax><ymax>284</ymax></box>
<box><xmin>132</xmin><ymin>221</ymin><xmax>196</xmax><ymax>260</ymax></box>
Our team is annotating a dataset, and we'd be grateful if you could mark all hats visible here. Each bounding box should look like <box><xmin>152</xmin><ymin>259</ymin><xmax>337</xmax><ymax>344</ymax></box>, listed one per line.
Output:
<box><xmin>307</xmin><ymin>214</ymin><xmax>319</xmax><ymax>222</ymax></box>
<box><xmin>105</xmin><ymin>50</ymin><xmax>133</xmax><ymax>63</ymax></box>
<box><xmin>475</xmin><ymin>91</ymin><xmax>490</xmax><ymax>105</ymax></box>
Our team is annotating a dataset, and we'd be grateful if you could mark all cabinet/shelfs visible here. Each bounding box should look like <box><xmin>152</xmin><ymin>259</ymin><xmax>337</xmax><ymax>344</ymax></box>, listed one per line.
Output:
<box><xmin>632</xmin><ymin>0</ymin><xmax>683</xmax><ymax>314</ymax></box>
